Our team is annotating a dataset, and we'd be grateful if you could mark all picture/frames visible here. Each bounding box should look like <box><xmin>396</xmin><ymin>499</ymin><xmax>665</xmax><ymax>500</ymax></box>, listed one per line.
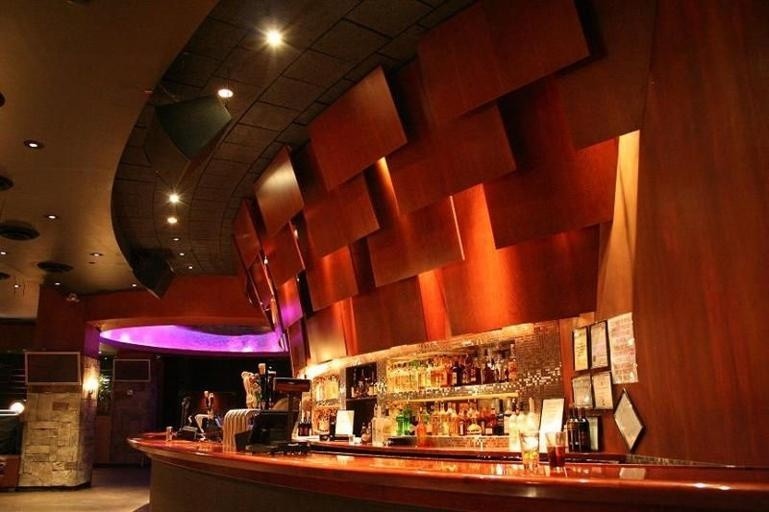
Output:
<box><xmin>571</xmin><ymin>320</ymin><xmax>645</xmax><ymax>453</ymax></box>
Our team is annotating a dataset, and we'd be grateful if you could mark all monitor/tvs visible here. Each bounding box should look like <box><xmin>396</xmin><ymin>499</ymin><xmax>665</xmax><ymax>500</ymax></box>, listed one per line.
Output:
<box><xmin>249</xmin><ymin>409</ymin><xmax>300</xmax><ymax>445</ymax></box>
<box><xmin>23</xmin><ymin>351</ymin><xmax>82</xmax><ymax>386</ymax></box>
<box><xmin>112</xmin><ymin>359</ymin><xmax>151</xmax><ymax>382</ymax></box>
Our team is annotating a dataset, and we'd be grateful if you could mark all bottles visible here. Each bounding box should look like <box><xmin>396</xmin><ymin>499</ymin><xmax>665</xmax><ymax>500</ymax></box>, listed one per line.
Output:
<box><xmin>386</xmin><ymin>351</ymin><xmax>506</xmax><ymax>394</ymax></box>
<box><xmin>567</xmin><ymin>408</ymin><xmax>590</xmax><ymax>452</ymax></box>
<box><xmin>298</xmin><ymin>410</ymin><xmax>313</xmax><ymax>436</ymax></box>
<box><xmin>504</xmin><ymin>397</ymin><xmax>526</xmax><ymax>449</ymax></box>
<box><xmin>394</xmin><ymin>402</ymin><xmax>498</xmax><ymax>436</ymax></box>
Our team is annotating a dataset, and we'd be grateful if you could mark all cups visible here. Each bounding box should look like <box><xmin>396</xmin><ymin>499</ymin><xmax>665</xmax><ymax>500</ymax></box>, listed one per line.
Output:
<box><xmin>349</xmin><ymin>435</ymin><xmax>355</xmax><ymax>444</ymax></box>
<box><xmin>544</xmin><ymin>432</ymin><xmax>567</xmax><ymax>473</ymax></box>
<box><xmin>518</xmin><ymin>432</ymin><xmax>540</xmax><ymax>472</ymax></box>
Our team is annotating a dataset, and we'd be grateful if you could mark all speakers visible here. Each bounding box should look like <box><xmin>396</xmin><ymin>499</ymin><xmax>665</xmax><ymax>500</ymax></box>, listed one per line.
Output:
<box><xmin>131</xmin><ymin>260</ymin><xmax>176</xmax><ymax>300</ymax></box>
<box><xmin>153</xmin><ymin>95</ymin><xmax>232</xmax><ymax>161</ymax></box>
